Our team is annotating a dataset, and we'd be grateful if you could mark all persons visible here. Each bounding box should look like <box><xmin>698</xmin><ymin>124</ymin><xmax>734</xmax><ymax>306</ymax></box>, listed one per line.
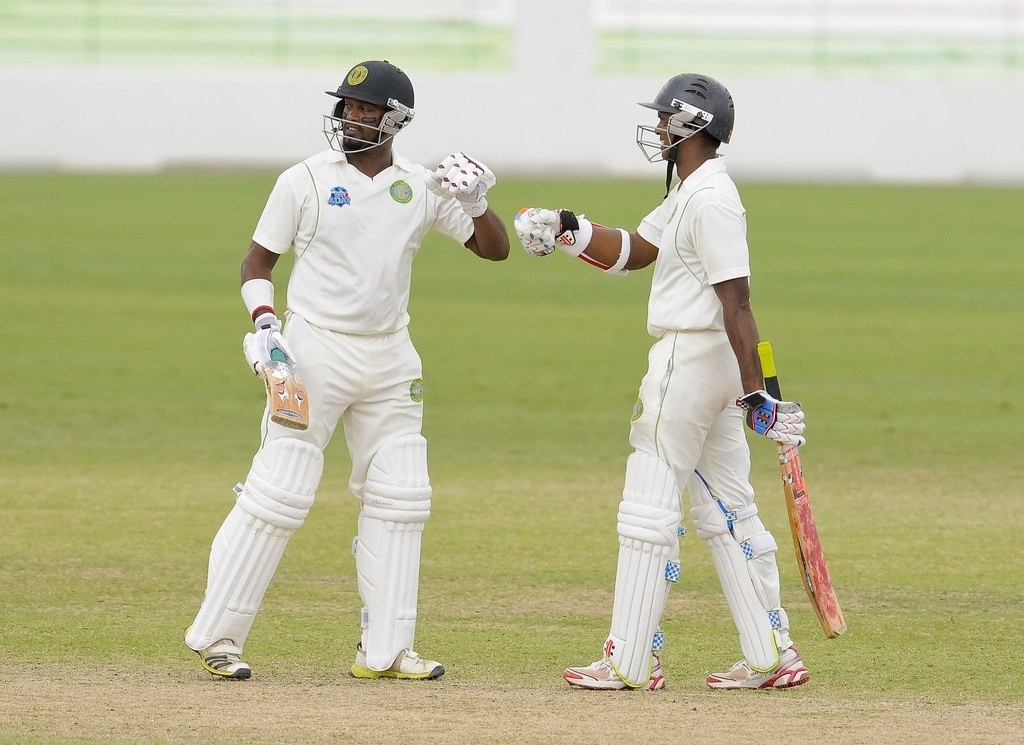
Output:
<box><xmin>185</xmin><ymin>59</ymin><xmax>507</xmax><ymax>680</ymax></box>
<box><xmin>513</xmin><ymin>72</ymin><xmax>807</xmax><ymax>690</ymax></box>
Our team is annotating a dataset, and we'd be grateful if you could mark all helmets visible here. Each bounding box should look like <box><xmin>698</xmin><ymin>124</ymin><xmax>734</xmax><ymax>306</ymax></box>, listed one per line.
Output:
<box><xmin>637</xmin><ymin>72</ymin><xmax>736</xmax><ymax>143</ymax></box>
<box><xmin>325</xmin><ymin>59</ymin><xmax>415</xmax><ymax>135</ymax></box>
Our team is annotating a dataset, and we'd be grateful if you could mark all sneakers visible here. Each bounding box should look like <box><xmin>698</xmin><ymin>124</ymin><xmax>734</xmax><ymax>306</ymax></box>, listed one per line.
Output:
<box><xmin>707</xmin><ymin>647</ymin><xmax>809</xmax><ymax>690</ymax></box>
<box><xmin>348</xmin><ymin>642</ymin><xmax>445</xmax><ymax>681</ymax></box>
<box><xmin>183</xmin><ymin>626</ymin><xmax>252</xmax><ymax>679</ymax></box>
<box><xmin>563</xmin><ymin>638</ymin><xmax>666</xmax><ymax>692</ymax></box>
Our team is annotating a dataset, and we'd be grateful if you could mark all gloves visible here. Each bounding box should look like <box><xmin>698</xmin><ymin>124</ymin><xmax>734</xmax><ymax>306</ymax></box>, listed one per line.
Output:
<box><xmin>243</xmin><ymin>313</ymin><xmax>296</xmax><ymax>381</ymax></box>
<box><xmin>423</xmin><ymin>152</ymin><xmax>498</xmax><ymax>219</ymax></box>
<box><xmin>736</xmin><ymin>388</ymin><xmax>806</xmax><ymax>447</ymax></box>
<box><xmin>513</xmin><ymin>206</ymin><xmax>594</xmax><ymax>259</ymax></box>
